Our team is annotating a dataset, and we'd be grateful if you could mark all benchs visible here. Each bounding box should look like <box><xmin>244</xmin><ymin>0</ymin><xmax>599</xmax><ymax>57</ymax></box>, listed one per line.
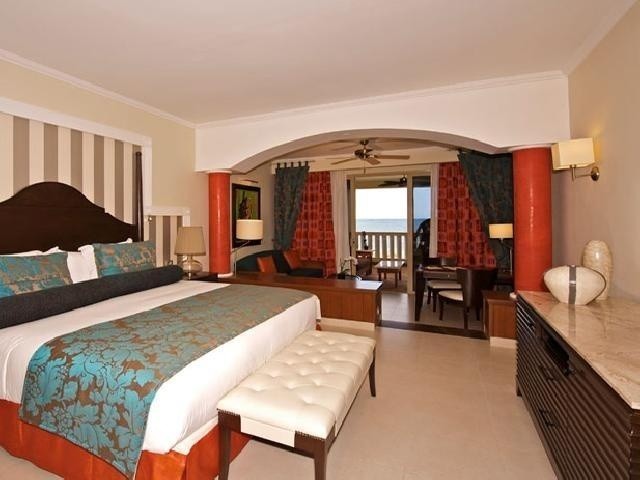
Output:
<box><xmin>215</xmin><ymin>327</ymin><xmax>378</xmax><ymax>479</ymax></box>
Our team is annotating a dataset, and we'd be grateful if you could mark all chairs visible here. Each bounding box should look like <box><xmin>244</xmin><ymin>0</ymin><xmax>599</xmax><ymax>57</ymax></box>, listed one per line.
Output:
<box><xmin>356</xmin><ymin>250</ymin><xmax>373</xmax><ymax>274</ymax></box>
<box><xmin>421</xmin><ymin>256</ymin><xmax>462</xmax><ymax>311</ymax></box>
<box><xmin>438</xmin><ymin>262</ymin><xmax>494</xmax><ymax>331</ymax></box>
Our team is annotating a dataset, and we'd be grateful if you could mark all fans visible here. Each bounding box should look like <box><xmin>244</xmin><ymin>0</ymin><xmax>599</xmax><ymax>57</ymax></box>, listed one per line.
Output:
<box><xmin>318</xmin><ymin>139</ymin><xmax>411</xmax><ymax>171</ymax></box>
<box><xmin>378</xmin><ymin>172</ymin><xmax>415</xmax><ymax>189</ymax></box>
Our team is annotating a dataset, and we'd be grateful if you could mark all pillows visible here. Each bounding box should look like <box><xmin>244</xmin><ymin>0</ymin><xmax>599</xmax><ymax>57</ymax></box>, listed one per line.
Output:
<box><xmin>0</xmin><ymin>250</ymin><xmax>72</xmax><ymax>300</ymax></box>
<box><xmin>284</xmin><ymin>250</ymin><xmax>301</xmax><ymax>268</ymax></box>
<box><xmin>0</xmin><ymin>264</ymin><xmax>185</xmax><ymax>329</ymax></box>
<box><xmin>94</xmin><ymin>238</ymin><xmax>156</xmax><ymax>279</ymax></box>
<box><xmin>258</xmin><ymin>256</ymin><xmax>278</xmax><ymax>274</ymax></box>
<box><xmin>58</xmin><ymin>248</ymin><xmax>86</xmax><ymax>285</ymax></box>
<box><xmin>79</xmin><ymin>238</ymin><xmax>134</xmax><ymax>278</ymax></box>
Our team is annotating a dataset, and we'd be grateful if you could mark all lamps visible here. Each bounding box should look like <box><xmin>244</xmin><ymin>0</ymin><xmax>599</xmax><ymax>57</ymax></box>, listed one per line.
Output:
<box><xmin>487</xmin><ymin>222</ymin><xmax>514</xmax><ymax>275</ymax></box>
<box><xmin>228</xmin><ymin>218</ymin><xmax>264</xmax><ymax>254</ymax></box>
<box><xmin>360</xmin><ymin>155</ymin><xmax>368</xmax><ymax>160</ymax></box>
<box><xmin>175</xmin><ymin>224</ymin><xmax>206</xmax><ymax>278</ymax></box>
<box><xmin>551</xmin><ymin>135</ymin><xmax>599</xmax><ymax>181</ymax></box>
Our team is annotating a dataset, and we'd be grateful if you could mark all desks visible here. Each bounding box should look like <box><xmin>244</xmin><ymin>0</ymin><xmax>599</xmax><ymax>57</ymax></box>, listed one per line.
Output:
<box><xmin>328</xmin><ymin>274</ymin><xmax>362</xmax><ymax>281</ymax></box>
<box><xmin>374</xmin><ymin>258</ymin><xmax>406</xmax><ymax>288</ymax></box>
<box><xmin>413</xmin><ymin>266</ymin><xmax>459</xmax><ymax>322</ymax></box>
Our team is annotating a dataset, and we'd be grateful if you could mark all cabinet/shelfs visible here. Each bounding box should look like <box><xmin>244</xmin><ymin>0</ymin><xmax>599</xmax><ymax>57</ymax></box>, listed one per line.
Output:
<box><xmin>512</xmin><ymin>287</ymin><xmax>637</xmax><ymax>480</ymax></box>
<box><xmin>477</xmin><ymin>289</ymin><xmax>517</xmax><ymax>347</ymax></box>
<box><xmin>221</xmin><ymin>271</ymin><xmax>382</xmax><ymax>327</ymax></box>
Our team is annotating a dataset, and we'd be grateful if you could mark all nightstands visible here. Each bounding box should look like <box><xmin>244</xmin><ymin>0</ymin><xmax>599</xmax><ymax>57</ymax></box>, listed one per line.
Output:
<box><xmin>179</xmin><ymin>268</ymin><xmax>218</xmax><ymax>283</ymax></box>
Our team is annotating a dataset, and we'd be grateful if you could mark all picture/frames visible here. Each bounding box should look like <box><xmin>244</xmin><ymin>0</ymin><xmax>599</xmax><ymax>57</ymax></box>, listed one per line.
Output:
<box><xmin>230</xmin><ymin>181</ymin><xmax>261</xmax><ymax>246</ymax></box>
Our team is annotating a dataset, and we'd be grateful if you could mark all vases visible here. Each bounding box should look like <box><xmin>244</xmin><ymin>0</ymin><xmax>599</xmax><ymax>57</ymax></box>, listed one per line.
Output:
<box><xmin>585</xmin><ymin>240</ymin><xmax>614</xmax><ymax>299</ymax></box>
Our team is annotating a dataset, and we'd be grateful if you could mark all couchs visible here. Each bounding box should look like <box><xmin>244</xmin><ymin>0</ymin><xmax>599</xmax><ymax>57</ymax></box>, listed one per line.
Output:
<box><xmin>233</xmin><ymin>248</ymin><xmax>327</xmax><ymax>279</ymax></box>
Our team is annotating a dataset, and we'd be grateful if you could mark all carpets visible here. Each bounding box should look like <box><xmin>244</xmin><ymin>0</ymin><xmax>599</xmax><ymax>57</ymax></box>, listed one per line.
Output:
<box><xmin>361</xmin><ymin>263</ymin><xmax>408</xmax><ymax>294</ymax></box>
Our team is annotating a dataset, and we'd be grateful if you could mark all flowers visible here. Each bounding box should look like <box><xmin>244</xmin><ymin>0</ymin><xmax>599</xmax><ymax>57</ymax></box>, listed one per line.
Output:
<box><xmin>344</xmin><ymin>254</ymin><xmax>359</xmax><ymax>276</ymax></box>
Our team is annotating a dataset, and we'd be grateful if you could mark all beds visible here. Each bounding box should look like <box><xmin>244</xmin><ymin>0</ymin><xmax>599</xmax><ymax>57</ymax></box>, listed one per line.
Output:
<box><xmin>0</xmin><ymin>179</ymin><xmax>324</xmax><ymax>479</ymax></box>
